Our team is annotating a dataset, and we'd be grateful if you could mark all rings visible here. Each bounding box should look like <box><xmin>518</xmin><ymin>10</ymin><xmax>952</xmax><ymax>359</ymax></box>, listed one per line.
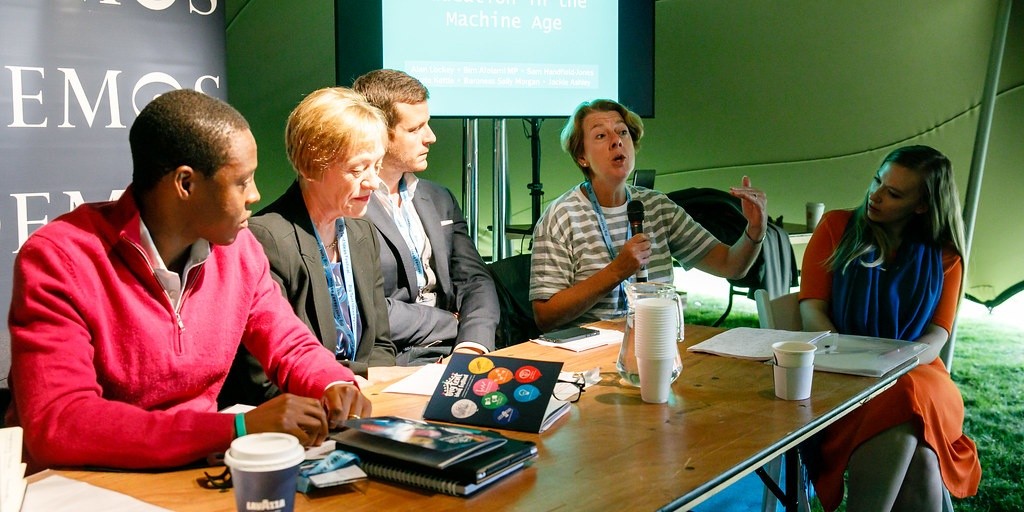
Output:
<box><xmin>349</xmin><ymin>414</ymin><xmax>360</xmax><ymax>419</ymax></box>
<box><xmin>753</xmin><ymin>194</ymin><xmax>758</xmax><ymax>199</ymax></box>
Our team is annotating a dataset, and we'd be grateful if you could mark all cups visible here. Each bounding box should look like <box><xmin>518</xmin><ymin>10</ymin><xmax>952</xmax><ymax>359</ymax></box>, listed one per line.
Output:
<box><xmin>806</xmin><ymin>202</ymin><xmax>825</xmax><ymax>230</ymax></box>
<box><xmin>635</xmin><ymin>298</ymin><xmax>676</xmax><ymax>403</ymax></box>
<box><xmin>773</xmin><ymin>341</ymin><xmax>817</xmax><ymax>401</ymax></box>
<box><xmin>223</xmin><ymin>432</ymin><xmax>305</xmax><ymax>512</ymax></box>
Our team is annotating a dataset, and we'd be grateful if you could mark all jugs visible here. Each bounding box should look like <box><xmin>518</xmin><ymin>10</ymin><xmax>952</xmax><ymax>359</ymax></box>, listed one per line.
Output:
<box><xmin>617</xmin><ymin>282</ymin><xmax>684</xmax><ymax>387</ymax></box>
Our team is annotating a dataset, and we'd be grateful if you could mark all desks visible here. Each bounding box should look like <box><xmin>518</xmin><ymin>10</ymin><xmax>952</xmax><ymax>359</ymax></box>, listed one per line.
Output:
<box><xmin>487</xmin><ymin>223</ymin><xmax>814</xmax><ymax>257</ymax></box>
<box><xmin>23</xmin><ymin>321</ymin><xmax>919</xmax><ymax>511</ymax></box>
<box><xmin>712</xmin><ymin>221</ymin><xmax>816</xmax><ymax>325</ymax></box>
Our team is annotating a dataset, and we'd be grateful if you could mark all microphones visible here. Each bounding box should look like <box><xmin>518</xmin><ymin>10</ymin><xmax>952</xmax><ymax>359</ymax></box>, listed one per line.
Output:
<box><xmin>627</xmin><ymin>199</ymin><xmax>648</xmax><ymax>284</ymax></box>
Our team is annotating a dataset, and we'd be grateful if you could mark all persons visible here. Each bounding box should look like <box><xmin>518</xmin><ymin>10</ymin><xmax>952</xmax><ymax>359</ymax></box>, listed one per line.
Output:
<box><xmin>219</xmin><ymin>88</ymin><xmax>397</xmax><ymax>407</ymax></box>
<box><xmin>352</xmin><ymin>70</ymin><xmax>501</xmax><ymax>366</ymax></box>
<box><xmin>6</xmin><ymin>88</ymin><xmax>373</xmax><ymax>474</ymax></box>
<box><xmin>530</xmin><ymin>99</ymin><xmax>767</xmax><ymax>330</ymax></box>
<box><xmin>799</xmin><ymin>146</ymin><xmax>982</xmax><ymax>512</ymax></box>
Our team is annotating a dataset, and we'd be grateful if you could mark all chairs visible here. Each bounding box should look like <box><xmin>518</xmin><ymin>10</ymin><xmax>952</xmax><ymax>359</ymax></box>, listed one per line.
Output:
<box><xmin>487</xmin><ymin>253</ymin><xmax>547</xmax><ymax>348</ymax></box>
<box><xmin>754</xmin><ymin>289</ymin><xmax>811</xmax><ymax>512</ymax></box>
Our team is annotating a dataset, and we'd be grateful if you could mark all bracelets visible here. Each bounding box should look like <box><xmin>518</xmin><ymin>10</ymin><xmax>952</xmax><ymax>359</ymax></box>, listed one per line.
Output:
<box><xmin>743</xmin><ymin>230</ymin><xmax>766</xmax><ymax>244</ymax></box>
<box><xmin>235</xmin><ymin>412</ymin><xmax>246</xmax><ymax>438</ymax></box>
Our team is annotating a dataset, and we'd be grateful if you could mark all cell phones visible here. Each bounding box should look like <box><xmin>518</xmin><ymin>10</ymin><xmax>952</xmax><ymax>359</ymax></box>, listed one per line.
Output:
<box><xmin>540</xmin><ymin>325</ymin><xmax>600</xmax><ymax>343</ymax></box>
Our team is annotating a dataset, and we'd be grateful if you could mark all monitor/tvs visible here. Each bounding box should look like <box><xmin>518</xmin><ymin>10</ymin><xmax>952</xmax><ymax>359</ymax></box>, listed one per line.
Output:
<box><xmin>335</xmin><ymin>0</ymin><xmax>656</xmax><ymax>120</ymax></box>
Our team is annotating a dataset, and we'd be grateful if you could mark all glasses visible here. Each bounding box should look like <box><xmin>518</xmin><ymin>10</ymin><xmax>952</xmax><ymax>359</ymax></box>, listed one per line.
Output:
<box><xmin>554</xmin><ymin>375</ymin><xmax>584</xmax><ymax>403</ymax></box>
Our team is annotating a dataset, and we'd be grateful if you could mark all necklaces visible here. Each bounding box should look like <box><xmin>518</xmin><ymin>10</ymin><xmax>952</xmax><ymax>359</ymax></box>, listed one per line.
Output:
<box><xmin>325</xmin><ymin>240</ymin><xmax>337</xmax><ymax>250</ymax></box>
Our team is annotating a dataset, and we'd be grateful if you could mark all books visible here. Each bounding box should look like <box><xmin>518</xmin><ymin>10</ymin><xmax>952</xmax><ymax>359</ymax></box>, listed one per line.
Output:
<box><xmin>687</xmin><ymin>327</ymin><xmax>831</xmax><ymax>360</ymax></box>
<box><xmin>423</xmin><ymin>352</ymin><xmax>571</xmax><ymax>433</ymax></box>
<box><xmin>329</xmin><ymin>416</ymin><xmax>538</xmax><ymax>497</ymax></box>
